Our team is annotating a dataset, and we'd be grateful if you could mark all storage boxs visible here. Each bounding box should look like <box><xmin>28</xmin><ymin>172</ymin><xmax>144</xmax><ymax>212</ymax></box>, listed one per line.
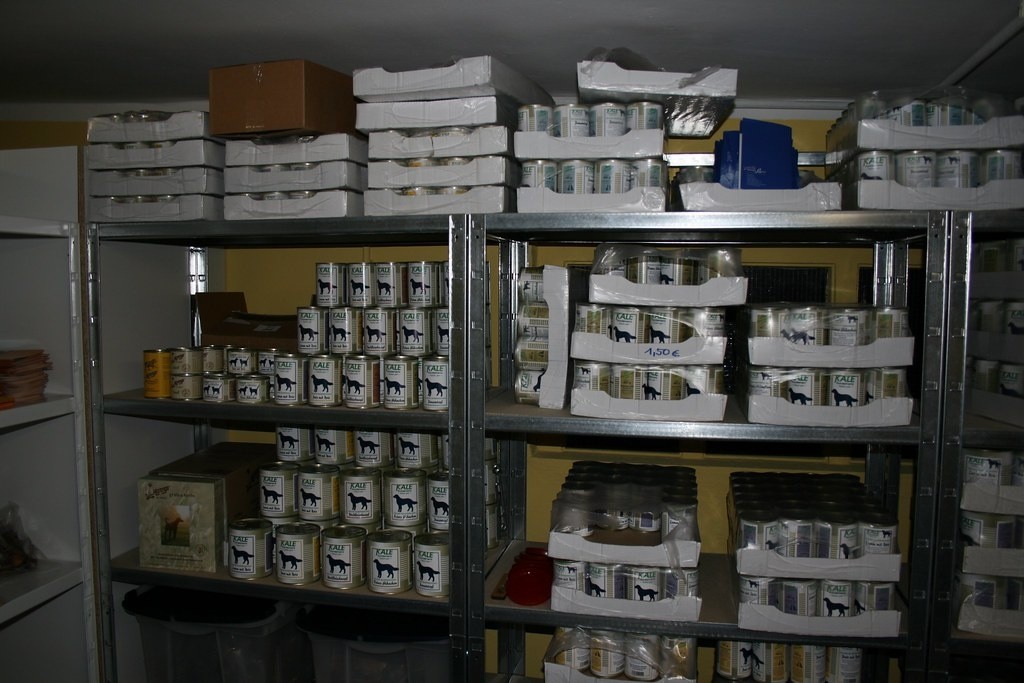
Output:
<box><xmin>195</xmin><ymin>292</ymin><xmax>298</xmax><ymax>353</ymax></box>
<box><xmin>224</xmin><ymin>161</ymin><xmax>368</xmax><ymax>194</ymax></box>
<box><xmin>87</xmin><ymin>140</ymin><xmax>224</xmax><ymax>170</ymax></box>
<box><xmin>225</xmin><ymin>132</ymin><xmax>369</xmax><ymax>167</ymax></box>
<box><xmin>351</xmin><ymin>56</ymin><xmax>1024</xmax><ymax>683</ymax></box>
<box><xmin>223</xmin><ymin>190</ymin><xmax>364</xmax><ymax>220</ymax></box>
<box><xmin>87</xmin><ymin>194</ymin><xmax>224</xmax><ymax>222</ymax></box>
<box><xmin>87</xmin><ymin>165</ymin><xmax>223</xmax><ymax>198</ymax></box>
<box><xmin>294</xmin><ymin>604</ymin><xmax>450</xmax><ymax>683</ymax></box>
<box><xmin>137</xmin><ymin>441</ymin><xmax>277</xmax><ymax>575</ymax></box>
<box><xmin>122</xmin><ymin>585</ymin><xmax>314</xmax><ymax>683</ymax></box>
<box><xmin>209</xmin><ymin>59</ymin><xmax>364</xmax><ymax>136</ymax></box>
<box><xmin>86</xmin><ymin>110</ymin><xmax>225</xmax><ymax>146</ymax></box>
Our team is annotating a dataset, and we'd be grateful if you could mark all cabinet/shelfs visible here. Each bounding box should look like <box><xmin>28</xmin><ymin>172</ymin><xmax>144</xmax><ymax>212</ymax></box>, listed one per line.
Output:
<box><xmin>466</xmin><ymin>211</ymin><xmax>949</xmax><ymax>683</ymax></box>
<box><xmin>924</xmin><ymin>211</ymin><xmax>1024</xmax><ymax>683</ymax></box>
<box><xmin>0</xmin><ymin>215</ymin><xmax>101</xmax><ymax>683</ymax></box>
<box><xmin>83</xmin><ymin>213</ymin><xmax>510</xmax><ymax>683</ymax></box>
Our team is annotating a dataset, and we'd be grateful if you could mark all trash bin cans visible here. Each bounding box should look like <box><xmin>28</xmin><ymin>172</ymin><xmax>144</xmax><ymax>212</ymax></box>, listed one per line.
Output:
<box><xmin>121</xmin><ymin>587</ymin><xmax>454</xmax><ymax>683</ymax></box>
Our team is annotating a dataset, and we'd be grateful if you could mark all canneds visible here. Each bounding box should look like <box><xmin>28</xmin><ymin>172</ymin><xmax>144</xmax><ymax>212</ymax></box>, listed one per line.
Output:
<box><xmin>226</xmin><ymin>426</ymin><xmax>500</xmax><ymax>599</ymax></box>
<box><xmin>91</xmin><ymin>89</ymin><xmax>1024</xmax><ymax>203</ymax></box>
<box><xmin>964</xmin><ymin>237</ymin><xmax>1024</xmax><ymax>400</ymax></box>
<box><xmin>552</xmin><ymin>625</ymin><xmax>861</xmax><ymax>683</ymax></box>
<box><xmin>512</xmin><ymin>247</ymin><xmax>727</xmax><ymax>406</ymax></box>
<box><xmin>955</xmin><ymin>448</ymin><xmax>1024</xmax><ymax>627</ymax></box>
<box><xmin>551</xmin><ymin>459</ymin><xmax>899</xmax><ymax>618</ymax></box>
<box><xmin>742</xmin><ymin>301</ymin><xmax>908</xmax><ymax>408</ymax></box>
<box><xmin>143</xmin><ymin>260</ymin><xmax>452</xmax><ymax>413</ymax></box>
